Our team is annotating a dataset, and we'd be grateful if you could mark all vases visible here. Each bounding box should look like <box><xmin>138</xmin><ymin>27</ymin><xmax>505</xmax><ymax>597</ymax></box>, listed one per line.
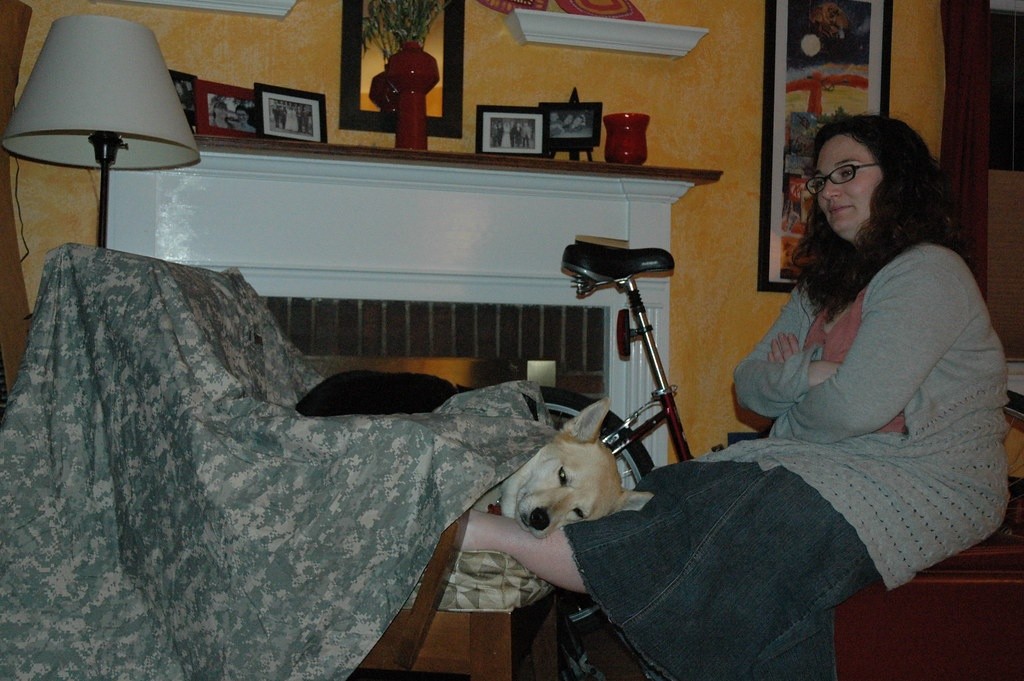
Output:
<box><xmin>368</xmin><ymin>63</ymin><xmax>394</xmax><ymax>117</ymax></box>
<box><xmin>168</xmin><ymin>69</ymin><xmax>198</xmax><ymax>135</ymax></box>
<box><xmin>604</xmin><ymin>112</ymin><xmax>650</xmax><ymax>163</ymax></box>
<box><xmin>386</xmin><ymin>39</ymin><xmax>441</xmax><ymax>152</ymax></box>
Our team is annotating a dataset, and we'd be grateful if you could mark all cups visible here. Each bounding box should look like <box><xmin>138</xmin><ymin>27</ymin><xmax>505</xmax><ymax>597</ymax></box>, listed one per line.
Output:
<box><xmin>602</xmin><ymin>112</ymin><xmax>651</xmax><ymax>165</ymax></box>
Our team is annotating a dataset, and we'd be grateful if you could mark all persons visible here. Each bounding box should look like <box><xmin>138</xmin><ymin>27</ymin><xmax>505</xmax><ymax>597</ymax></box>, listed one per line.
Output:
<box><xmin>491</xmin><ymin>120</ymin><xmax>534</xmax><ymax>148</ymax></box>
<box><xmin>458</xmin><ymin>113</ymin><xmax>1011</xmax><ymax>681</ymax></box>
<box><xmin>549</xmin><ymin>114</ymin><xmax>588</xmax><ymax>136</ymax></box>
<box><xmin>271</xmin><ymin>101</ymin><xmax>310</xmax><ymax>134</ymax></box>
<box><xmin>211</xmin><ymin>100</ymin><xmax>255</xmax><ymax>133</ymax></box>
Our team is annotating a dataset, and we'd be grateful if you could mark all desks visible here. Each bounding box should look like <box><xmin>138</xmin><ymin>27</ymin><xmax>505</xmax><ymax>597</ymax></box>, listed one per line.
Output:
<box><xmin>830</xmin><ymin>479</ymin><xmax>1024</xmax><ymax>681</ymax></box>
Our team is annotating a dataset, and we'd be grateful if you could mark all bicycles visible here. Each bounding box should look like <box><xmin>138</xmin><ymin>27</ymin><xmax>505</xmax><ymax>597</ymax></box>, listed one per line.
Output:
<box><xmin>522</xmin><ymin>245</ymin><xmax>1024</xmax><ymax>624</ymax></box>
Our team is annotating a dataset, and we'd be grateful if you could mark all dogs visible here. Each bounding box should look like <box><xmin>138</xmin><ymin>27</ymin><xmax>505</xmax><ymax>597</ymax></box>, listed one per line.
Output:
<box><xmin>470</xmin><ymin>395</ymin><xmax>655</xmax><ymax>547</ymax></box>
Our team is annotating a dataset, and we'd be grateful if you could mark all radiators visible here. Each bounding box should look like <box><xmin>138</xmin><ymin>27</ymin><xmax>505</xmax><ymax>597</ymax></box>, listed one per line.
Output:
<box><xmin>264</xmin><ymin>299</ymin><xmax>605</xmax><ymax>410</ymax></box>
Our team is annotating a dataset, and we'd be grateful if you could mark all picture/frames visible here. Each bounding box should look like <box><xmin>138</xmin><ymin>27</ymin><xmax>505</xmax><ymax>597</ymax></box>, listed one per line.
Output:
<box><xmin>541</xmin><ymin>102</ymin><xmax>603</xmax><ymax>150</ymax></box>
<box><xmin>475</xmin><ymin>104</ymin><xmax>548</xmax><ymax>156</ymax></box>
<box><xmin>340</xmin><ymin>0</ymin><xmax>464</xmax><ymax>138</ymax></box>
<box><xmin>253</xmin><ymin>82</ymin><xmax>329</xmax><ymax>142</ymax></box>
<box><xmin>194</xmin><ymin>80</ymin><xmax>255</xmax><ymax>139</ymax></box>
<box><xmin>760</xmin><ymin>0</ymin><xmax>895</xmax><ymax>298</ymax></box>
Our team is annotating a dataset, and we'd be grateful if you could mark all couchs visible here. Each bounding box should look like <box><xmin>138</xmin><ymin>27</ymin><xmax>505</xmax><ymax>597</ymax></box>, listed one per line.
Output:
<box><xmin>0</xmin><ymin>245</ymin><xmax>538</xmax><ymax>681</ymax></box>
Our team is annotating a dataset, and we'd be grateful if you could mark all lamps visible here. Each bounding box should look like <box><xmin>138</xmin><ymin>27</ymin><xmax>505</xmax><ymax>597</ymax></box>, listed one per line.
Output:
<box><xmin>0</xmin><ymin>14</ymin><xmax>201</xmax><ymax>253</ymax></box>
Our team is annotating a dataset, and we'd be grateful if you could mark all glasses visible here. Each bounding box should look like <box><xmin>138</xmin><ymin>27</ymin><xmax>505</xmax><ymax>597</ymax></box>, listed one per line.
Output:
<box><xmin>805</xmin><ymin>163</ymin><xmax>878</xmax><ymax>195</ymax></box>
